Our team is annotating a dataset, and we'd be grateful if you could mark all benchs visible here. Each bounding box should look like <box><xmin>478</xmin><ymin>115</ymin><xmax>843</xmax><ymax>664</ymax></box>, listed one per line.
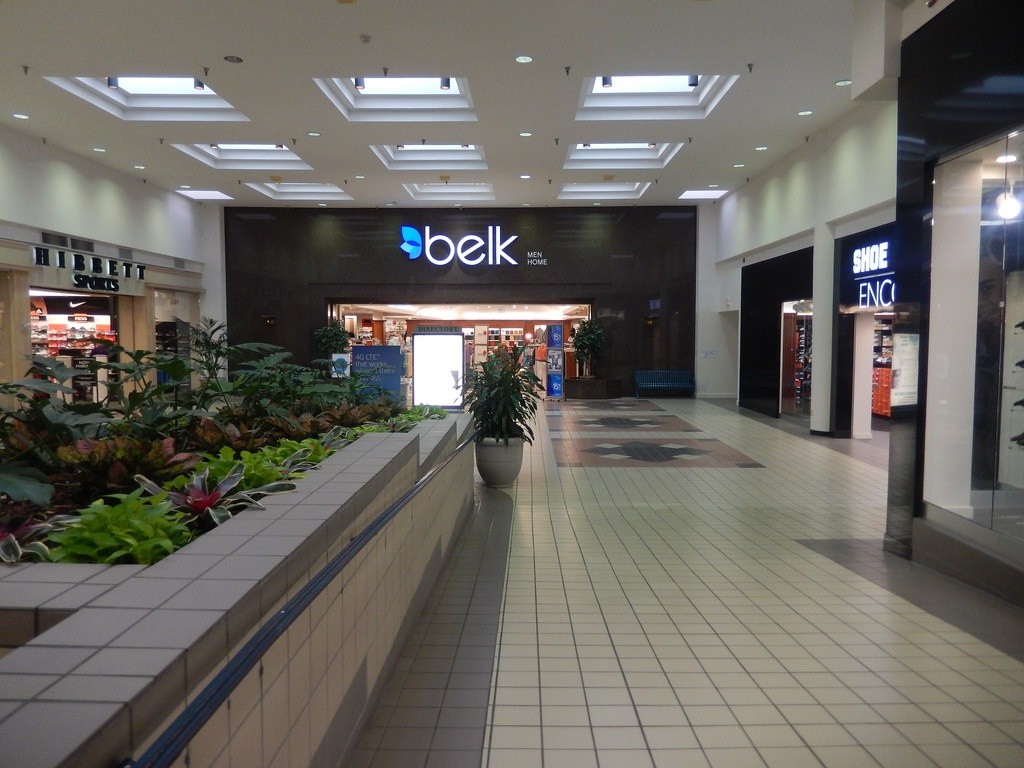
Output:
<box><xmin>633</xmin><ymin>369</ymin><xmax>695</xmax><ymax>399</ymax></box>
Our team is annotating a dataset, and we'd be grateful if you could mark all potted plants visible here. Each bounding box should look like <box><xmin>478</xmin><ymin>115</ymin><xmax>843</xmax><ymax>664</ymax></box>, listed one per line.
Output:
<box><xmin>564</xmin><ymin>318</ymin><xmax>608</xmax><ymax>398</ymax></box>
<box><xmin>453</xmin><ymin>340</ymin><xmax>551</xmax><ymax>488</ymax></box>
<box><xmin>312</xmin><ymin>318</ymin><xmax>350</xmax><ymax>385</ymax></box>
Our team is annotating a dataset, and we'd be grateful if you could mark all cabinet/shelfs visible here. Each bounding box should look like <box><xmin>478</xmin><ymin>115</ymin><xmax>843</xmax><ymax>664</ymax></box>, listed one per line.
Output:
<box><xmin>784</xmin><ymin>313</ymin><xmax>812</xmax><ymax>413</ymax></box>
<box><xmin>156</xmin><ymin>321</ymin><xmax>191</xmax><ymax>426</ymax></box>
<box><xmin>31</xmin><ymin>329</ymin><xmax>116</xmax><ymax>356</ymax></box>
<box><xmin>872</xmin><ymin>311</ymin><xmax>892</xmax><ymax>417</ymax></box>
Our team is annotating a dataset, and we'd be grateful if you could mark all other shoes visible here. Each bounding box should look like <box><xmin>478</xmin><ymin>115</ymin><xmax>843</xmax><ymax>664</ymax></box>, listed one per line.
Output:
<box><xmin>33</xmin><ymin>326</ymin><xmax>118</xmax><ymax>357</ymax></box>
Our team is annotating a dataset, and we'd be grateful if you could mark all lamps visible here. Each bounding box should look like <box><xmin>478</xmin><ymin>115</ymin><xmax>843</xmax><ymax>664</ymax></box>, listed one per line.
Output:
<box><xmin>108</xmin><ymin>78</ymin><xmax>118</xmax><ymax>88</ymax></box>
<box><xmin>354</xmin><ymin>78</ymin><xmax>365</xmax><ymax>89</ymax></box>
<box><xmin>647</xmin><ymin>317</ymin><xmax>653</xmax><ymax>326</ymax></box>
<box><xmin>688</xmin><ymin>76</ymin><xmax>699</xmax><ymax>85</ymax></box>
<box><xmin>602</xmin><ymin>77</ymin><xmax>611</xmax><ymax>86</ymax></box>
<box><xmin>194</xmin><ymin>78</ymin><xmax>205</xmax><ymax>90</ymax></box>
<box><xmin>440</xmin><ymin>78</ymin><xmax>450</xmax><ymax>89</ymax></box>
<box><xmin>996</xmin><ymin>182</ymin><xmax>1020</xmax><ymax>219</ymax></box>
<box><xmin>262</xmin><ymin>315</ymin><xmax>275</xmax><ymax>325</ymax></box>
